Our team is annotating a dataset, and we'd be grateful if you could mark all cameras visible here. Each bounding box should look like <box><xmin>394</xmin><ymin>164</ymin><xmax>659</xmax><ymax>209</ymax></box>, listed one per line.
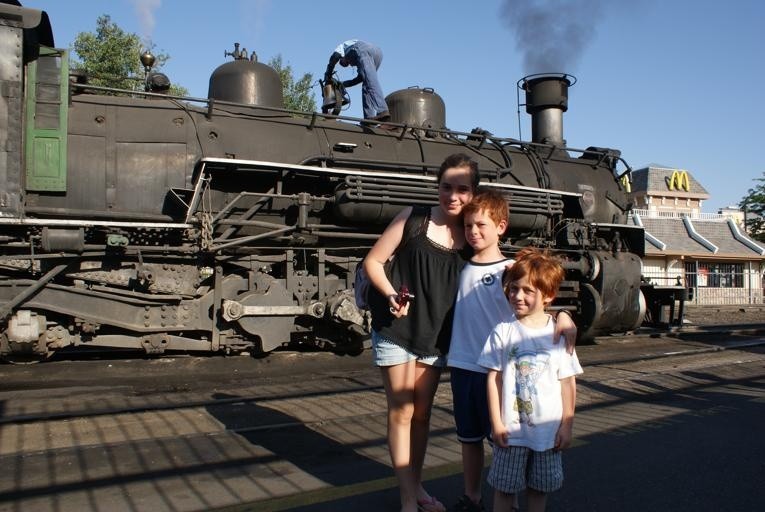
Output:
<box><xmin>396</xmin><ymin>284</ymin><xmax>416</xmax><ymax>312</ymax></box>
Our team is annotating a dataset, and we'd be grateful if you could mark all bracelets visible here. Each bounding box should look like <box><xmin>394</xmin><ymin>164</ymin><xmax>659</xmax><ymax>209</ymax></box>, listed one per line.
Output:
<box><xmin>554</xmin><ymin>307</ymin><xmax>574</xmax><ymax>322</ymax></box>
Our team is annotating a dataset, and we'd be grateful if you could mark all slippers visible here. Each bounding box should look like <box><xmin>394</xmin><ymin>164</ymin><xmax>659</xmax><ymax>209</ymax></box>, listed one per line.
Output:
<box><xmin>417</xmin><ymin>495</ymin><xmax>447</xmax><ymax>512</ymax></box>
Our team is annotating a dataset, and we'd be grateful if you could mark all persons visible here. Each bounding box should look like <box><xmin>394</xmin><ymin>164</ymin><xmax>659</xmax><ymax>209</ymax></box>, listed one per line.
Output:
<box><xmin>362</xmin><ymin>152</ymin><xmax>579</xmax><ymax>512</ymax></box>
<box><xmin>324</xmin><ymin>39</ymin><xmax>392</xmax><ymax>129</ymax></box>
<box><xmin>446</xmin><ymin>192</ymin><xmax>517</xmax><ymax>511</ymax></box>
<box><xmin>475</xmin><ymin>246</ymin><xmax>585</xmax><ymax>511</ymax></box>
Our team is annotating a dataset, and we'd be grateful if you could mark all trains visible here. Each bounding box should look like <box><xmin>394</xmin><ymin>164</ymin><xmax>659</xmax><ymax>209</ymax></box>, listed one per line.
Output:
<box><xmin>0</xmin><ymin>0</ymin><xmax>651</xmax><ymax>365</ymax></box>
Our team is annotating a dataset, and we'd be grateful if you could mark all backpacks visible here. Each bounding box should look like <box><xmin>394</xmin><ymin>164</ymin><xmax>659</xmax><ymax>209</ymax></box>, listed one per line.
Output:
<box><xmin>355</xmin><ymin>205</ymin><xmax>429</xmax><ymax>311</ymax></box>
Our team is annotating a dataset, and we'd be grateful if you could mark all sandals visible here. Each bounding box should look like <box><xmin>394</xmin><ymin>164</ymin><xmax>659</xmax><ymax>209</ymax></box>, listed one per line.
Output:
<box><xmin>447</xmin><ymin>491</ymin><xmax>486</xmax><ymax>512</ymax></box>
